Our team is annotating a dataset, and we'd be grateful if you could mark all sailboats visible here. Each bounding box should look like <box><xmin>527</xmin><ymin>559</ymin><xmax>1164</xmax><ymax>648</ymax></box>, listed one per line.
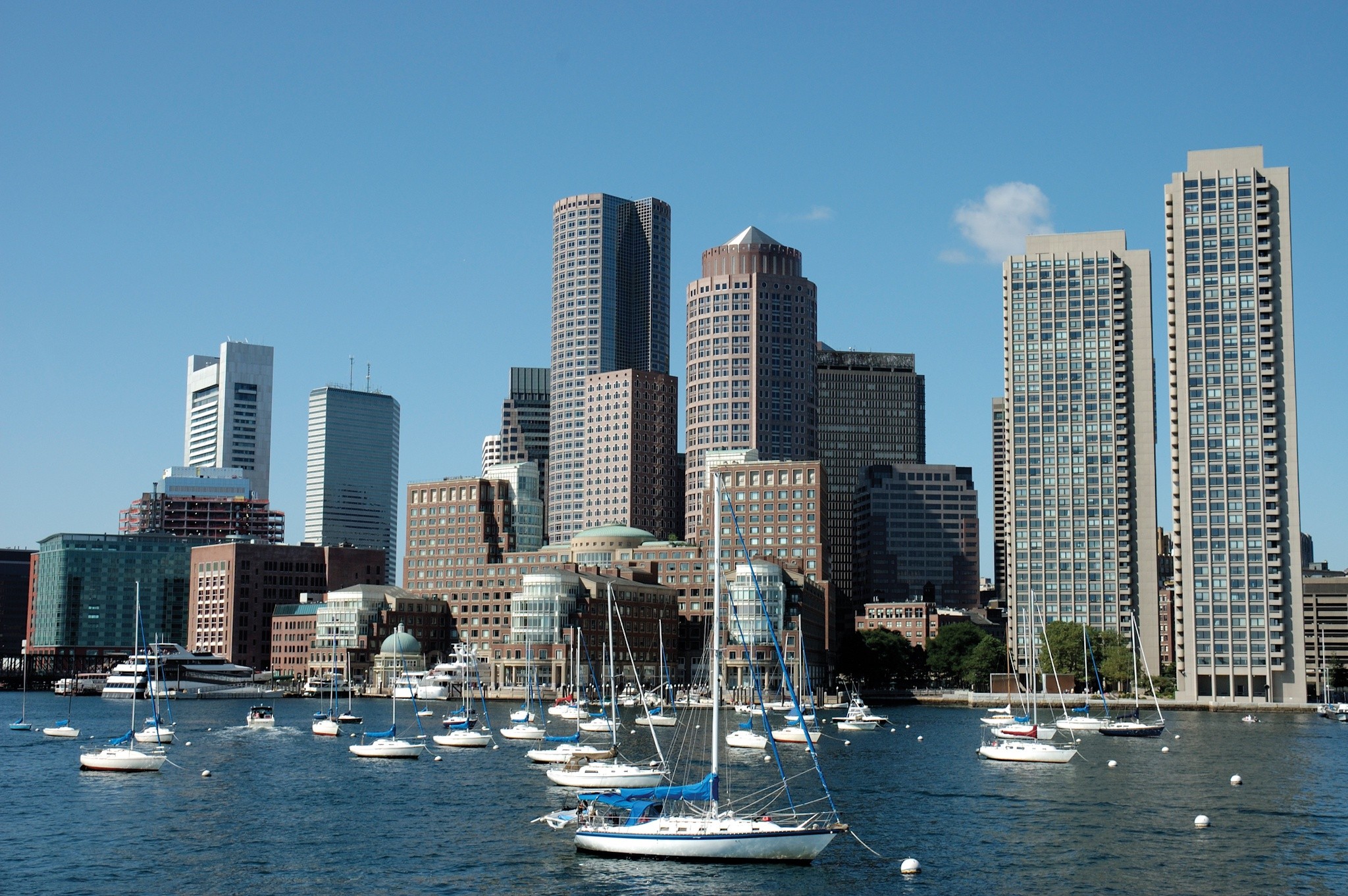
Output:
<box><xmin>283</xmin><ymin>615</ymin><xmax>365</xmax><ymax>737</ymax></box>
<box><xmin>725</xmin><ymin>621</ymin><xmax>769</xmax><ymax>749</ymax></box>
<box><xmin>1056</xmin><ymin>622</ymin><xmax>1115</xmax><ymax>730</ymax></box>
<box><xmin>526</xmin><ymin>470</ymin><xmax>849</xmax><ymax>866</ymax></box>
<box><xmin>8</xmin><ymin>646</ymin><xmax>32</xmax><ymax>730</ymax></box>
<box><xmin>349</xmin><ymin>627</ymin><xmax>427</xmax><ymax>759</ymax></box>
<box><xmin>499</xmin><ymin>617</ymin><xmax>716</xmax><ymax>763</ymax></box>
<box><xmin>43</xmin><ymin>670</ymin><xmax>81</xmax><ymax>737</ymax></box>
<box><xmin>975</xmin><ymin>589</ymin><xmax>1081</xmax><ymax>763</ymax></box>
<box><xmin>979</xmin><ymin>608</ymin><xmax>1058</xmax><ymax>740</ymax></box>
<box><xmin>133</xmin><ymin>632</ymin><xmax>175</xmax><ymax>744</ymax></box>
<box><xmin>79</xmin><ymin>581</ymin><xmax>171</xmax><ymax>772</ymax></box>
<box><xmin>1316</xmin><ymin>627</ymin><xmax>1348</xmax><ymax>722</ymax></box>
<box><xmin>547</xmin><ymin>578</ymin><xmax>672</xmax><ymax>788</ymax></box>
<box><xmin>1098</xmin><ymin>609</ymin><xmax>1165</xmax><ymax>737</ymax></box>
<box><xmin>770</xmin><ymin>615</ymin><xmax>824</xmax><ymax>743</ymax></box>
<box><xmin>391</xmin><ymin>632</ymin><xmax>493</xmax><ymax>748</ymax></box>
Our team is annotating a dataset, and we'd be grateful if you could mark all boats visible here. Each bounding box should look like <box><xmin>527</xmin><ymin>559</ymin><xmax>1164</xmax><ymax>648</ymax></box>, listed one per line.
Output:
<box><xmin>831</xmin><ymin>679</ymin><xmax>889</xmax><ymax>730</ymax></box>
<box><xmin>246</xmin><ymin>702</ymin><xmax>275</xmax><ymax>729</ymax></box>
<box><xmin>544</xmin><ymin>808</ymin><xmax>589</xmax><ymax>829</ymax></box>
<box><xmin>54</xmin><ymin>632</ymin><xmax>285</xmax><ymax>700</ymax></box>
<box><xmin>1242</xmin><ymin>716</ymin><xmax>1261</xmax><ymax>723</ymax></box>
